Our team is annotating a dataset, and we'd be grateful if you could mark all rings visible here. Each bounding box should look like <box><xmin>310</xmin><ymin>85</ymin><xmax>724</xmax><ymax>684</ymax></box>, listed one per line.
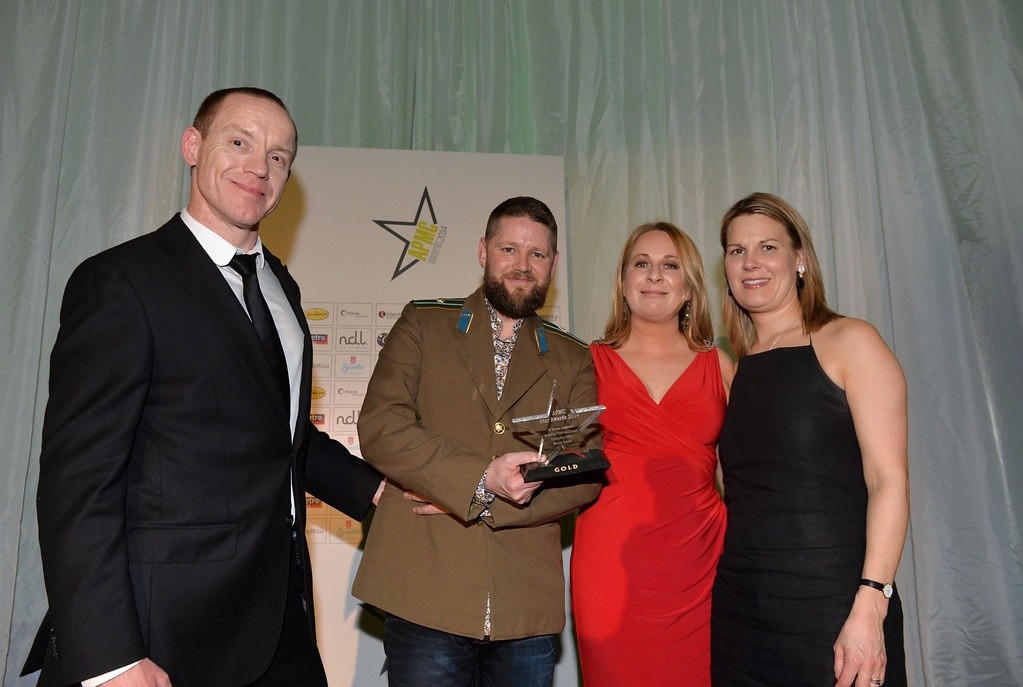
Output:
<box><xmin>871</xmin><ymin>678</ymin><xmax>882</xmax><ymax>684</ymax></box>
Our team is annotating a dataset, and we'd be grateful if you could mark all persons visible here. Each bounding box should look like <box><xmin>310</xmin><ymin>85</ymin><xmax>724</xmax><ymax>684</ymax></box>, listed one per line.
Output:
<box><xmin>350</xmin><ymin>196</ymin><xmax>603</xmax><ymax>687</ymax></box>
<box><xmin>710</xmin><ymin>193</ymin><xmax>910</xmax><ymax>687</ymax></box>
<box><xmin>19</xmin><ymin>87</ymin><xmax>387</xmax><ymax>687</ymax></box>
<box><xmin>569</xmin><ymin>221</ymin><xmax>734</xmax><ymax>687</ymax></box>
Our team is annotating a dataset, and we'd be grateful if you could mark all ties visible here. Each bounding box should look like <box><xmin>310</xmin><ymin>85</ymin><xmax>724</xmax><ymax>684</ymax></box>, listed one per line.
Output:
<box><xmin>228</xmin><ymin>252</ymin><xmax>291</xmax><ymax>425</ymax></box>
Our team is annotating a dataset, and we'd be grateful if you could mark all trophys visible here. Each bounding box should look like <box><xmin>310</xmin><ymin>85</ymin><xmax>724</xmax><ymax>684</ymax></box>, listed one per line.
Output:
<box><xmin>512</xmin><ymin>379</ymin><xmax>612</xmax><ymax>482</ymax></box>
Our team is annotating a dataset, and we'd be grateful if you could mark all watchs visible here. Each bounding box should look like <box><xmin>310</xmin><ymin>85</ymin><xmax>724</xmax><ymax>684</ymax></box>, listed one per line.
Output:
<box><xmin>859</xmin><ymin>579</ymin><xmax>893</xmax><ymax>598</ymax></box>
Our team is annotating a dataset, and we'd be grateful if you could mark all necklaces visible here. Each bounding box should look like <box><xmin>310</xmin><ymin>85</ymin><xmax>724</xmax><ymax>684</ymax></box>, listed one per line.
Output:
<box><xmin>767</xmin><ymin>324</ymin><xmax>792</xmax><ymax>351</ymax></box>
